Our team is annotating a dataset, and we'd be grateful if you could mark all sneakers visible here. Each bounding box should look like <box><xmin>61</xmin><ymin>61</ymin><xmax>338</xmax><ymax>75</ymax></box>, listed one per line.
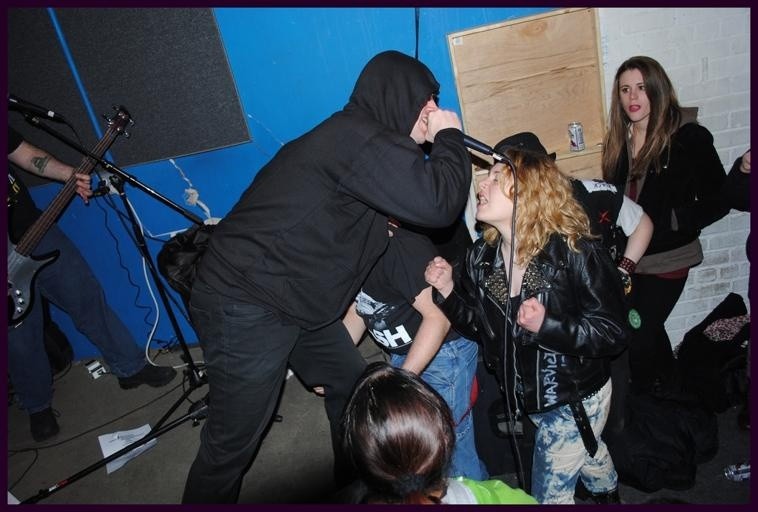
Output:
<box><xmin>118</xmin><ymin>364</ymin><xmax>176</xmax><ymax>390</ymax></box>
<box><xmin>30</xmin><ymin>407</ymin><xmax>59</xmax><ymax>443</ymax></box>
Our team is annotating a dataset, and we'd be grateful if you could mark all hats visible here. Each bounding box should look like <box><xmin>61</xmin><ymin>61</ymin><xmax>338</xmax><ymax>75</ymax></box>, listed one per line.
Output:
<box><xmin>493</xmin><ymin>132</ymin><xmax>556</xmax><ymax>164</ymax></box>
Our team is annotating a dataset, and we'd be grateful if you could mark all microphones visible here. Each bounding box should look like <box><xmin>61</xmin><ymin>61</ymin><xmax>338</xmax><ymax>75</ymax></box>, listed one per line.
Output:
<box><xmin>461</xmin><ymin>133</ymin><xmax>506</xmax><ymax>168</ymax></box>
<box><xmin>7</xmin><ymin>93</ymin><xmax>69</xmax><ymax>126</ymax></box>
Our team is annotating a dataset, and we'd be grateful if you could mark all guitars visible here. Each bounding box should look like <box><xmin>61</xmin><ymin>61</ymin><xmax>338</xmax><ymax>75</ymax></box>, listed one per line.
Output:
<box><xmin>6</xmin><ymin>105</ymin><xmax>135</xmax><ymax>326</ymax></box>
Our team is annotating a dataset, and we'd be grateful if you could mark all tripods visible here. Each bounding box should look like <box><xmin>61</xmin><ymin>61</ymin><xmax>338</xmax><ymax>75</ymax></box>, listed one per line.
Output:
<box><xmin>143</xmin><ymin>140</ymin><xmax>283</xmax><ymax>445</ymax></box>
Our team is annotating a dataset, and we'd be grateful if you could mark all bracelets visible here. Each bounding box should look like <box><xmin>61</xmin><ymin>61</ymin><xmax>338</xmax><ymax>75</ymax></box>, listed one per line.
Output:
<box><xmin>618</xmin><ymin>256</ymin><xmax>637</xmax><ymax>275</ymax></box>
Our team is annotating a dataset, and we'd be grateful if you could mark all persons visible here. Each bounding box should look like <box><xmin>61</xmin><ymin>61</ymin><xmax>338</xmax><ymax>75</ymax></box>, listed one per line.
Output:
<box><xmin>726</xmin><ymin>149</ymin><xmax>752</xmax><ymax>430</ymax></box>
<box><xmin>5</xmin><ymin>125</ymin><xmax>179</xmax><ymax>443</ymax></box>
<box><xmin>421</xmin><ymin>144</ymin><xmax>632</xmax><ymax>506</ymax></box>
<box><xmin>179</xmin><ymin>48</ymin><xmax>474</xmax><ymax>506</ymax></box>
<box><xmin>312</xmin><ymin>212</ymin><xmax>492</xmax><ymax>486</ymax></box>
<box><xmin>600</xmin><ymin>54</ymin><xmax>734</xmax><ymax>393</ymax></box>
<box><xmin>493</xmin><ymin>131</ymin><xmax>654</xmax><ymax>500</ymax></box>
<box><xmin>338</xmin><ymin>360</ymin><xmax>539</xmax><ymax>506</ymax></box>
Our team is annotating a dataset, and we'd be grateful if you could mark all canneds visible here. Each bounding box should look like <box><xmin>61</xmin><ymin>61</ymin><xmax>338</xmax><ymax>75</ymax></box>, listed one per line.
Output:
<box><xmin>724</xmin><ymin>463</ymin><xmax>751</xmax><ymax>483</ymax></box>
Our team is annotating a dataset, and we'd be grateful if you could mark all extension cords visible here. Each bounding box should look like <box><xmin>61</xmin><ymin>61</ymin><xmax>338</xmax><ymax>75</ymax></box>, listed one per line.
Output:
<box><xmin>86</xmin><ymin>358</ymin><xmax>105</xmax><ymax>379</ymax></box>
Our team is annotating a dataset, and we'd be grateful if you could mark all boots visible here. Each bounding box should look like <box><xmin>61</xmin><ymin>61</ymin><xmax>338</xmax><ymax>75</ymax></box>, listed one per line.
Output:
<box><xmin>590</xmin><ymin>487</ymin><xmax>621</xmax><ymax>505</ymax></box>
